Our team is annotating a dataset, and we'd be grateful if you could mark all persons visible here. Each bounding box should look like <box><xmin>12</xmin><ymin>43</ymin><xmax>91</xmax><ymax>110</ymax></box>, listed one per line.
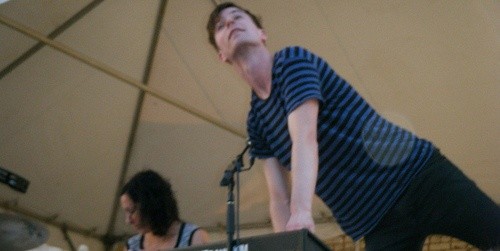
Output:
<box><xmin>206</xmin><ymin>2</ymin><xmax>500</xmax><ymax>251</ymax></box>
<box><xmin>118</xmin><ymin>169</ymin><xmax>209</xmax><ymax>251</ymax></box>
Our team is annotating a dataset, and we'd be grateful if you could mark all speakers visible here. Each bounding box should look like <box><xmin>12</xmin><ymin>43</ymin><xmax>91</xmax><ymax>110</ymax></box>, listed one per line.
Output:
<box><xmin>168</xmin><ymin>228</ymin><xmax>329</xmax><ymax>251</ymax></box>
